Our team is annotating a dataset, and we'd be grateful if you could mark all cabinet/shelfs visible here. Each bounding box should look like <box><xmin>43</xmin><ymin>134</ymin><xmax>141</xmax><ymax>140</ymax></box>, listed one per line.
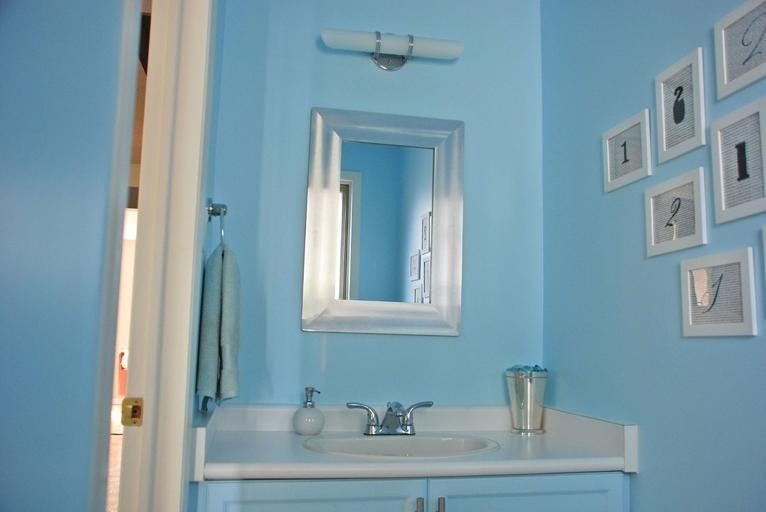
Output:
<box><xmin>197</xmin><ymin>470</ymin><xmax>631</xmax><ymax>511</ymax></box>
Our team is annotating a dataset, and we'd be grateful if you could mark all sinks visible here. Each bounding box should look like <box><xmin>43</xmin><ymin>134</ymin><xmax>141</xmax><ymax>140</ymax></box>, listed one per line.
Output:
<box><xmin>303</xmin><ymin>430</ymin><xmax>499</xmax><ymax>462</ymax></box>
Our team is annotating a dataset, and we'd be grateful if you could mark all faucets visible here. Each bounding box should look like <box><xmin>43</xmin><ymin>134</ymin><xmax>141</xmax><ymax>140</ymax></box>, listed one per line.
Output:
<box><xmin>378</xmin><ymin>400</ymin><xmax>409</xmax><ymax>435</ymax></box>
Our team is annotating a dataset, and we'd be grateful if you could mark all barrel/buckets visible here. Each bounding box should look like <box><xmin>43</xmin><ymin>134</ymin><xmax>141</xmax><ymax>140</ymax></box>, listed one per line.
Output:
<box><xmin>504</xmin><ymin>370</ymin><xmax>550</xmax><ymax>430</ymax></box>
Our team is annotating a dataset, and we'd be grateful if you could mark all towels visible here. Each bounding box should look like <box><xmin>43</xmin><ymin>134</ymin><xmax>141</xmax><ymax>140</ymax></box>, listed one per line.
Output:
<box><xmin>195</xmin><ymin>244</ymin><xmax>240</xmax><ymax>416</ymax></box>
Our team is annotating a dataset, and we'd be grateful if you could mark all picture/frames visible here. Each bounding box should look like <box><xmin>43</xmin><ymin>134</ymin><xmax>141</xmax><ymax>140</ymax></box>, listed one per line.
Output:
<box><xmin>601</xmin><ymin>108</ymin><xmax>654</xmax><ymax>194</ymax></box>
<box><xmin>654</xmin><ymin>48</ymin><xmax>708</xmax><ymax>164</ymax></box>
<box><xmin>643</xmin><ymin>167</ymin><xmax>708</xmax><ymax>256</ymax></box>
<box><xmin>679</xmin><ymin>247</ymin><xmax>758</xmax><ymax>336</ymax></box>
<box><xmin>710</xmin><ymin>100</ymin><xmax>766</xmax><ymax>225</ymax></box>
<box><xmin>409</xmin><ymin>210</ymin><xmax>433</xmax><ymax>303</ymax></box>
<box><xmin>714</xmin><ymin>0</ymin><xmax>766</xmax><ymax>102</ymax></box>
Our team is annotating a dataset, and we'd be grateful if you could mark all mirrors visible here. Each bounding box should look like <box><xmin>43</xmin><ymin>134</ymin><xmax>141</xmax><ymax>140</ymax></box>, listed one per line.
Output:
<box><xmin>300</xmin><ymin>107</ymin><xmax>465</xmax><ymax>337</ymax></box>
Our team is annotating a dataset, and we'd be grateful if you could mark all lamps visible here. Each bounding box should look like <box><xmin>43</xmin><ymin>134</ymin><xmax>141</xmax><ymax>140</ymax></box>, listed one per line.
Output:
<box><xmin>320</xmin><ymin>29</ymin><xmax>465</xmax><ymax>70</ymax></box>
